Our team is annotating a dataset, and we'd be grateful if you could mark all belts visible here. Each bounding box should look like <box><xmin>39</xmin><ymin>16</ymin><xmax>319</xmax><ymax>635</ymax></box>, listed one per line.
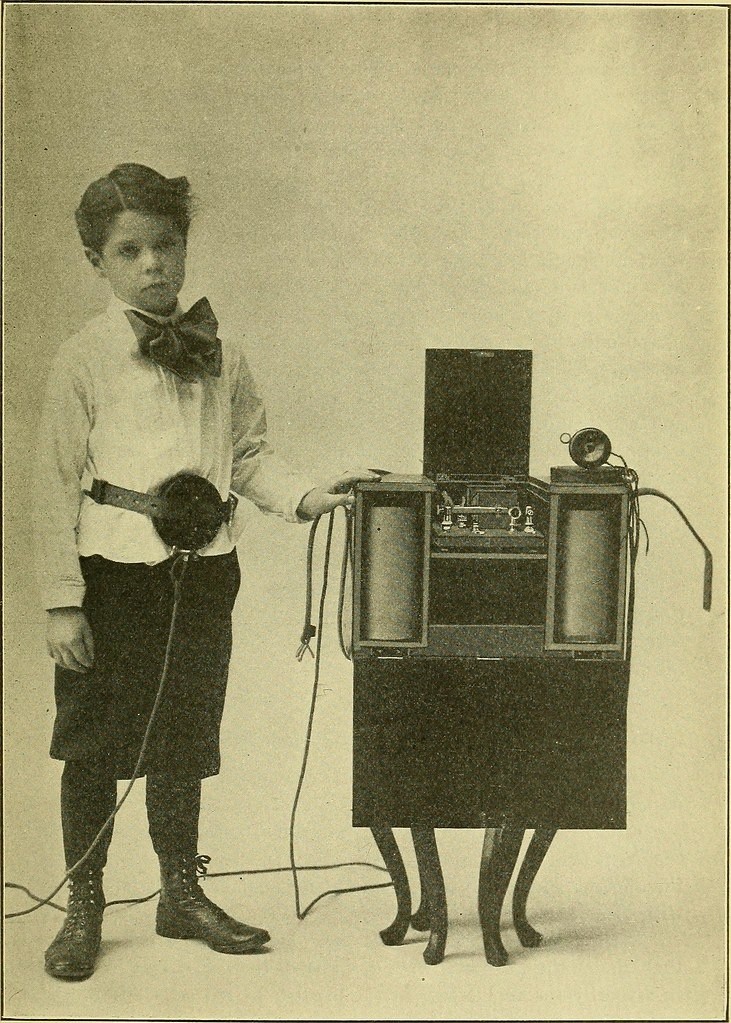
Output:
<box><xmin>89</xmin><ymin>477</ymin><xmax>237</xmax><ymax>525</ymax></box>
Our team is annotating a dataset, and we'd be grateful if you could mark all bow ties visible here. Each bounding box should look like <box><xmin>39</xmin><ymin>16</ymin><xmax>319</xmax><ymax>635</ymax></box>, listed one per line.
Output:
<box><xmin>121</xmin><ymin>297</ymin><xmax>223</xmax><ymax>383</ymax></box>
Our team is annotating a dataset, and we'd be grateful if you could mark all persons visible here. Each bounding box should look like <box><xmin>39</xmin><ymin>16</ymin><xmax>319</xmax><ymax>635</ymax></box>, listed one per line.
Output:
<box><xmin>31</xmin><ymin>161</ymin><xmax>382</xmax><ymax>980</ymax></box>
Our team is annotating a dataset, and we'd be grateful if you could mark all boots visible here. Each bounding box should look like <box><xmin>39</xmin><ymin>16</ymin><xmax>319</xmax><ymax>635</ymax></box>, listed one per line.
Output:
<box><xmin>44</xmin><ymin>867</ymin><xmax>106</xmax><ymax>977</ymax></box>
<box><xmin>156</xmin><ymin>852</ymin><xmax>272</xmax><ymax>953</ymax></box>
